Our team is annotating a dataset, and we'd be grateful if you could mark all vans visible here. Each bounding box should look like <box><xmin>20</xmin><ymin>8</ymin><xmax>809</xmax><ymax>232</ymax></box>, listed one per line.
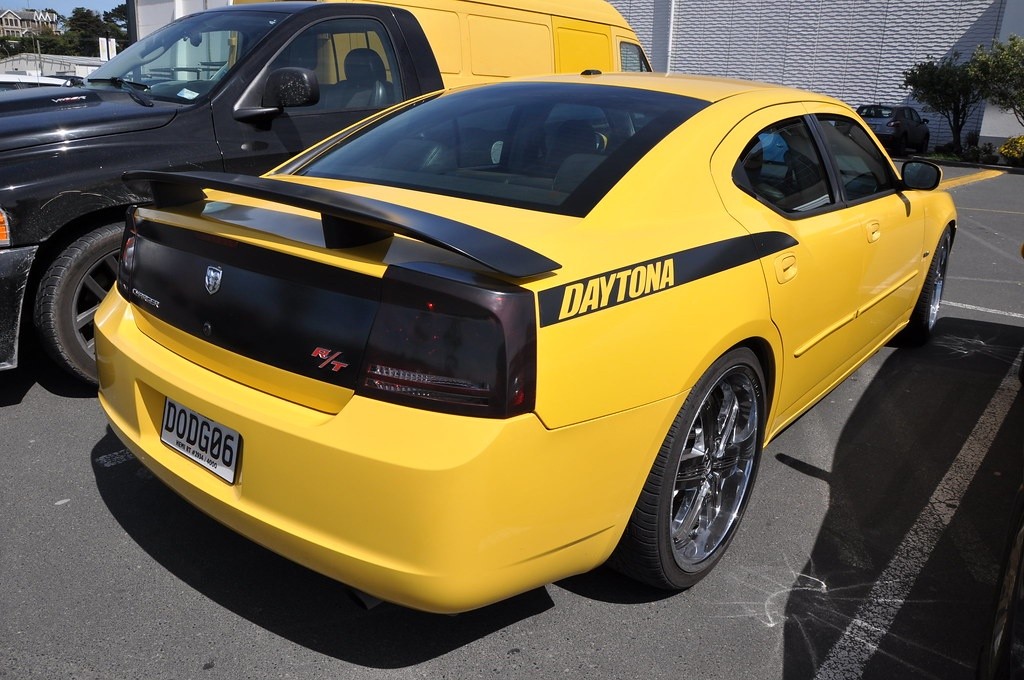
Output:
<box><xmin>227</xmin><ymin>0</ymin><xmax>655</xmax><ymax>90</ymax></box>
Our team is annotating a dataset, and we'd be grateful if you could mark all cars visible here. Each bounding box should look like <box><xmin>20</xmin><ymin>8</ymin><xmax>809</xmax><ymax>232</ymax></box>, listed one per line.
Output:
<box><xmin>855</xmin><ymin>101</ymin><xmax>931</xmax><ymax>157</ymax></box>
<box><xmin>90</xmin><ymin>71</ymin><xmax>960</xmax><ymax>616</ymax></box>
<box><xmin>0</xmin><ymin>73</ymin><xmax>88</xmax><ymax>92</ymax></box>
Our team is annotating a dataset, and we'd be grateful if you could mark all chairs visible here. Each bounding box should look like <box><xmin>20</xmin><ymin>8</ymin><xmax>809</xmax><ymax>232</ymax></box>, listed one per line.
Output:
<box><xmin>320</xmin><ymin>49</ymin><xmax>385</xmax><ymax>114</ymax></box>
<box><xmin>541</xmin><ymin>119</ymin><xmax>601</xmax><ymax>175</ymax></box>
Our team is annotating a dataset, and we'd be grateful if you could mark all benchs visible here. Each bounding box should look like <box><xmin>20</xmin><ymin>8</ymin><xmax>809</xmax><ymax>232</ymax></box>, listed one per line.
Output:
<box><xmin>390</xmin><ymin>138</ymin><xmax>608</xmax><ymax>195</ymax></box>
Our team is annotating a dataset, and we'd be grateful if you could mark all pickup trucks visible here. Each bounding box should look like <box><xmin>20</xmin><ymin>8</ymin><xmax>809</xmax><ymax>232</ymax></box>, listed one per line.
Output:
<box><xmin>0</xmin><ymin>0</ymin><xmax>633</xmax><ymax>388</ymax></box>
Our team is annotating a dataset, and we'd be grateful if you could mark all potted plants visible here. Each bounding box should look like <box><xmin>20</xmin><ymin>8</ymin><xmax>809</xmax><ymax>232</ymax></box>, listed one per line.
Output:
<box><xmin>982</xmin><ymin>142</ymin><xmax>999</xmax><ymax>163</ymax></box>
